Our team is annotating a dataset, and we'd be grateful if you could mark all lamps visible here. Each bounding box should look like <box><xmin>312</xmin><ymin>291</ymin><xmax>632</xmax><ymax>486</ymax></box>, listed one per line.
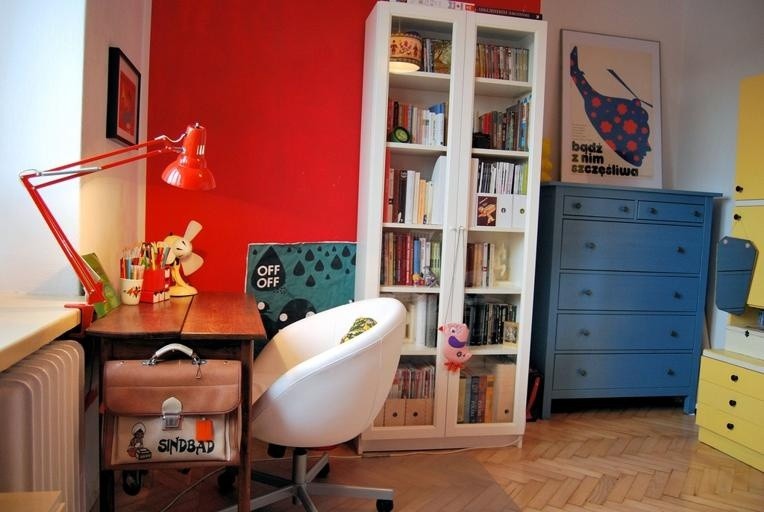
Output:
<box><xmin>17</xmin><ymin>122</ymin><xmax>217</xmax><ymax>333</ymax></box>
<box><xmin>389</xmin><ymin>18</ymin><xmax>423</xmax><ymax>72</ymax></box>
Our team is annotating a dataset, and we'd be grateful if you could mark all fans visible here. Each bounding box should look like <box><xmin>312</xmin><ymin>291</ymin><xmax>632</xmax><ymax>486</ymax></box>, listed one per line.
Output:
<box><xmin>156</xmin><ymin>220</ymin><xmax>205</xmax><ymax>298</ymax></box>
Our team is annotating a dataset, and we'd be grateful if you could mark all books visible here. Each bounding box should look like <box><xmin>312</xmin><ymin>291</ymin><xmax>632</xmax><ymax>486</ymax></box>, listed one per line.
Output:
<box><xmin>423</xmin><ymin>36</ymin><xmax>451</xmax><ymax>72</ymax></box>
<box><xmin>386</xmin><ymin>99</ymin><xmax>448</xmax><ymax>145</ymax></box>
<box><xmin>384</xmin><ymin>362</ymin><xmax>433</xmax><ymax>424</ymax></box>
<box><xmin>457</xmin><ymin>358</ymin><xmax>514</xmax><ymax>424</ymax></box>
<box><xmin>382</xmin><ymin>149</ymin><xmax>448</xmax><ymax>224</ymax></box>
<box><xmin>476</xmin><ymin>44</ymin><xmax>529</xmax><ymax>83</ymax></box>
<box><xmin>463</xmin><ymin>297</ymin><xmax>516</xmax><ymax>345</ymax></box>
<box><xmin>408</xmin><ymin>294</ymin><xmax>437</xmax><ymax>347</ymax></box>
<box><xmin>472</xmin><ymin>97</ymin><xmax>530</xmax><ymax>151</ymax></box>
<box><xmin>381</xmin><ymin>230</ymin><xmax>441</xmax><ymax>288</ymax></box>
<box><xmin>465</xmin><ymin>244</ymin><xmax>518</xmax><ymax>289</ymax></box>
<box><xmin>470</xmin><ymin>158</ymin><xmax>529</xmax><ymax>229</ymax></box>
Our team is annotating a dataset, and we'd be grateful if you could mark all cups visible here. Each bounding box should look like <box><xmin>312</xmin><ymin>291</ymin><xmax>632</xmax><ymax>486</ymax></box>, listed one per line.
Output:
<box><xmin>421</xmin><ymin>36</ymin><xmax>434</xmax><ymax>73</ymax></box>
<box><xmin>119</xmin><ymin>277</ymin><xmax>144</xmax><ymax>306</ymax></box>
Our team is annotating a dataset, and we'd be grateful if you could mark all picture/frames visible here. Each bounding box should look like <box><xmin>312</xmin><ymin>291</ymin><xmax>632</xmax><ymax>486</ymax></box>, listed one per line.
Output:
<box><xmin>559</xmin><ymin>29</ymin><xmax>664</xmax><ymax>190</ymax></box>
<box><xmin>106</xmin><ymin>46</ymin><xmax>141</xmax><ymax>150</ymax></box>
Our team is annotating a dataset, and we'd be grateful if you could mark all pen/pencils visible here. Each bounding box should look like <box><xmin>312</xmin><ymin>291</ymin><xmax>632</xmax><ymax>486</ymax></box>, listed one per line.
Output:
<box><xmin>142</xmin><ymin>242</ymin><xmax>171</xmax><ymax>267</ymax></box>
<box><xmin>121</xmin><ymin>247</ymin><xmax>145</xmax><ymax>279</ymax></box>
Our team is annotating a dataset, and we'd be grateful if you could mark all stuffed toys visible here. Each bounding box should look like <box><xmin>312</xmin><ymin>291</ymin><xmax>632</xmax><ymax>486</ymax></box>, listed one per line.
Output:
<box><xmin>436</xmin><ymin>322</ymin><xmax>476</xmax><ymax>373</ymax></box>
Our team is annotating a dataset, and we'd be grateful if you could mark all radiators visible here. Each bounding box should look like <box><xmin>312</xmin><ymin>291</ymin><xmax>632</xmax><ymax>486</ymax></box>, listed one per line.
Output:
<box><xmin>0</xmin><ymin>340</ymin><xmax>87</xmax><ymax>512</ymax></box>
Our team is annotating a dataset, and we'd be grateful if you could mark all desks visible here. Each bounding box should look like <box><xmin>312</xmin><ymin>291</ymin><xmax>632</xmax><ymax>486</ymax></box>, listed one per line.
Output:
<box><xmin>0</xmin><ymin>489</ymin><xmax>66</xmax><ymax>512</ymax></box>
<box><xmin>84</xmin><ymin>290</ymin><xmax>267</xmax><ymax>512</ymax></box>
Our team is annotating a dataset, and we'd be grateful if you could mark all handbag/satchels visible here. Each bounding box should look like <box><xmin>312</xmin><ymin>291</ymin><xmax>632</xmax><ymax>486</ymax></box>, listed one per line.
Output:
<box><xmin>100</xmin><ymin>345</ymin><xmax>244</xmax><ymax>468</ymax></box>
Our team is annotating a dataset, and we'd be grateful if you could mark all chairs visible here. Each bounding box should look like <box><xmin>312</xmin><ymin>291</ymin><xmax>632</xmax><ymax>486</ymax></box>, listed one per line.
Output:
<box><xmin>214</xmin><ymin>297</ymin><xmax>407</xmax><ymax>512</ymax></box>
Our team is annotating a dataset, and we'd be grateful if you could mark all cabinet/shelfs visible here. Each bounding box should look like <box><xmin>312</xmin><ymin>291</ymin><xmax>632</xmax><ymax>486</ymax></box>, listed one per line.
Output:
<box><xmin>529</xmin><ymin>182</ymin><xmax>724</xmax><ymax>421</ymax></box>
<box><xmin>353</xmin><ymin>1</ymin><xmax>549</xmax><ymax>457</ymax></box>
<box><xmin>693</xmin><ymin>345</ymin><xmax>764</xmax><ymax>473</ymax></box>
